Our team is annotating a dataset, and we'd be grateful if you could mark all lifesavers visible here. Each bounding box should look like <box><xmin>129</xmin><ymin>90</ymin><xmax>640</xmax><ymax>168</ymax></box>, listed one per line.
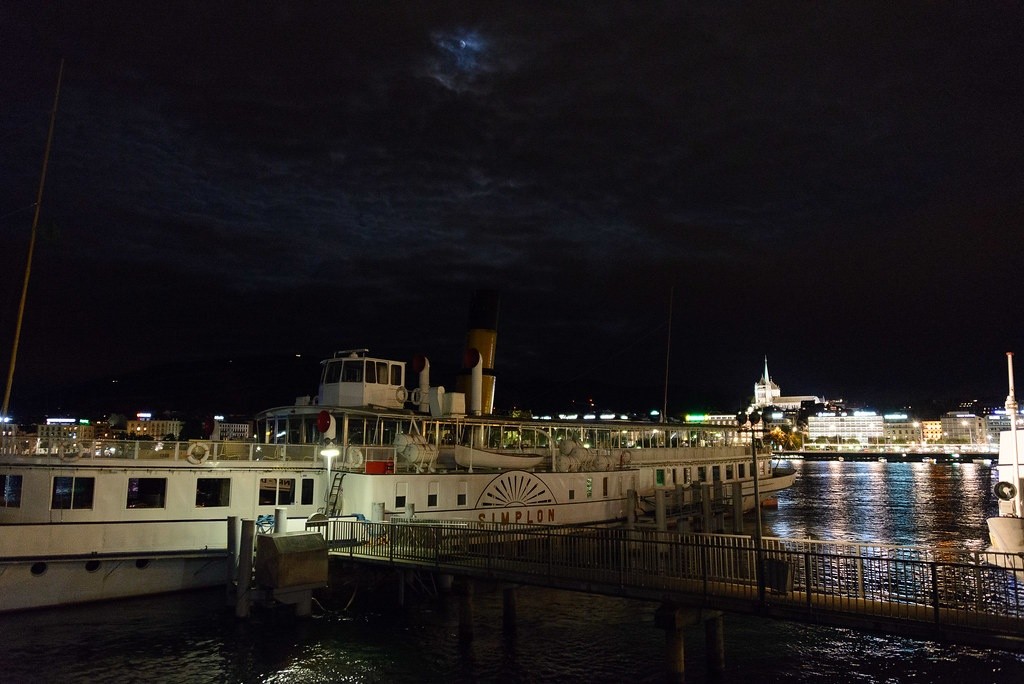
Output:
<box><xmin>395</xmin><ymin>386</ymin><xmax>408</xmax><ymax>403</ymax></box>
<box><xmin>994</xmin><ymin>482</ymin><xmax>1017</xmax><ymax>501</ymax></box>
<box><xmin>411</xmin><ymin>388</ymin><xmax>424</xmax><ymax>405</ymax></box>
<box><xmin>621</xmin><ymin>450</ymin><xmax>632</xmax><ymax>465</ymax></box>
<box><xmin>186</xmin><ymin>441</ymin><xmax>210</xmax><ymax>465</ymax></box>
<box><xmin>58</xmin><ymin>438</ymin><xmax>84</xmax><ymax>462</ymax></box>
<box><xmin>347</xmin><ymin>447</ymin><xmax>363</xmax><ymax>468</ymax></box>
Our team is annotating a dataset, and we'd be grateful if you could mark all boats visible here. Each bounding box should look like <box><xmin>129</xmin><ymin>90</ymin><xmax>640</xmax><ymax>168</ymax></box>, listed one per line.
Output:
<box><xmin>981</xmin><ymin>351</ymin><xmax>1024</xmax><ymax>581</ymax></box>
<box><xmin>0</xmin><ymin>344</ymin><xmax>804</xmax><ymax>623</ymax></box>
